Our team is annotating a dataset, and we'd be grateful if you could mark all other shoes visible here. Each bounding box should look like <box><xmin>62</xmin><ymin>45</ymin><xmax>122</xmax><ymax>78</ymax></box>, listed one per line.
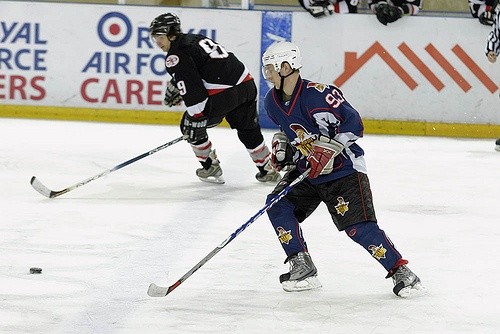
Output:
<box><xmin>495</xmin><ymin>139</ymin><xmax>500</xmax><ymax>151</ymax></box>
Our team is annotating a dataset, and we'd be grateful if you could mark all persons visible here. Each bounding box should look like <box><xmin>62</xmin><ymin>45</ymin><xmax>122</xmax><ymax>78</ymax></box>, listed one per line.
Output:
<box><xmin>148</xmin><ymin>12</ymin><xmax>281</xmax><ymax>184</ymax></box>
<box><xmin>468</xmin><ymin>0</ymin><xmax>500</xmax><ymax>63</ymax></box>
<box><xmin>298</xmin><ymin>0</ymin><xmax>424</xmax><ymax>26</ymax></box>
<box><xmin>261</xmin><ymin>40</ymin><xmax>420</xmax><ymax>297</ymax></box>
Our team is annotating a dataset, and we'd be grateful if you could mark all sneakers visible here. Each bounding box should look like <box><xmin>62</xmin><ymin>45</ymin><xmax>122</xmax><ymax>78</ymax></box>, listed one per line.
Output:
<box><xmin>196</xmin><ymin>149</ymin><xmax>225</xmax><ymax>183</ymax></box>
<box><xmin>385</xmin><ymin>262</ymin><xmax>427</xmax><ymax>298</ymax></box>
<box><xmin>255</xmin><ymin>159</ymin><xmax>283</xmax><ymax>185</ymax></box>
<box><xmin>279</xmin><ymin>241</ymin><xmax>323</xmax><ymax>292</ymax></box>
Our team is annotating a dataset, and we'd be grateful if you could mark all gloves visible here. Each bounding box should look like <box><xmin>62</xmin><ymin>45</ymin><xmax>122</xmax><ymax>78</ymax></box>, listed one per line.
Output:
<box><xmin>309</xmin><ymin>0</ymin><xmax>334</xmax><ymax>18</ymax></box>
<box><xmin>164</xmin><ymin>81</ymin><xmax>184</xmax><ymax>107</ymax></box>
<box><xmin>479</xmin><ymin>11</ymin><xmax>496</xmax><ymax>26</ymax></box>
<box><xmin>271</xmin><ymin>133</ymin><xmax>291</xmax><ymax>172</ymax></box>
<box><xmin>183</xmin><ymin>112</ymin><xmax>208</xmax><ymax>144</ymax></box>
<box><xmin>306</xmin><ymin>134</ymin><xmax>344</xmax><ymax>179</ymax></box>
<box><xmin>376</xmin><ymin>3</ymin><xmax>404</xmax><ymax>25</ymax></box>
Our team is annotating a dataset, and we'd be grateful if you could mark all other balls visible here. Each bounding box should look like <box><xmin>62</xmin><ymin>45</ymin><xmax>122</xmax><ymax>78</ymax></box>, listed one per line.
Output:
<box><xmin>30</xmin><ymin>268</ymin><xmax>43</xmax><ymax>274</ymax></box>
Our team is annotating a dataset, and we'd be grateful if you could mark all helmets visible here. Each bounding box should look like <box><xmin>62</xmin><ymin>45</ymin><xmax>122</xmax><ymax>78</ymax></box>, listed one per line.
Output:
<box><xmin>262</xmin><ymin>41</ymin><xmax>303</xmax><ymax>73</ymax></box>
<box><xmin>150</xmin><ymin>12</ymin><xmax>182</xmax><ymax>36</ymax></box>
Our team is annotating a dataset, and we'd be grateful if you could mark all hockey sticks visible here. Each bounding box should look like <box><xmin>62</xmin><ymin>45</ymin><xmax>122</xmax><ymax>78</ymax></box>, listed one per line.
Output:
<box><xmin>147</xmin><ymin>168</ymin><xmax>312</xmax><ymax>298</ymax></box>
<box><xmin>31</xmin><ymin>134</ymin><xmax>189</xmax><ymax>199</ymax></box>
